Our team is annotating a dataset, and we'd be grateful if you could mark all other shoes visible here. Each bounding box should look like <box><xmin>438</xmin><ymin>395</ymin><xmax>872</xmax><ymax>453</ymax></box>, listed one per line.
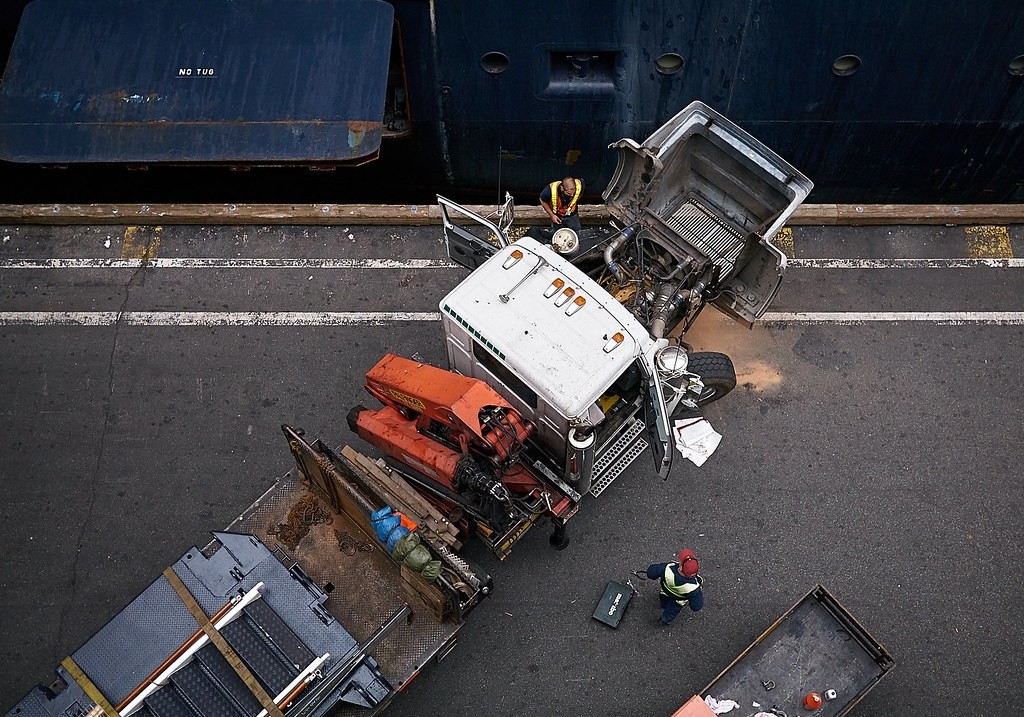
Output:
<box><xmin>657</xmin><ymin>619</ymin><xmax>669</xmax><ymax>626</ymax></box>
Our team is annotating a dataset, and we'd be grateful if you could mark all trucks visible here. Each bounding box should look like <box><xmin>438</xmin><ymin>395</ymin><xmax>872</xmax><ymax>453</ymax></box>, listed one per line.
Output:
<box><xmin>671</xmin><ymin>585</ymin><xmax>897</xmax><ymax>717</ymax></box>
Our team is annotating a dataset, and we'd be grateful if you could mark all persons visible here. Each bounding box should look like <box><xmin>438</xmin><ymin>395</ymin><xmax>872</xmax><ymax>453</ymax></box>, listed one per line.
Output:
<box><xmin>647</xmin><ymin>549</ymin><xmax>703</xmax><ymax>625</ymax></box>
<box><xmin>540</xmin><ymin>177</ymin><xmax>585</xmax><ymax>231</ymax></box>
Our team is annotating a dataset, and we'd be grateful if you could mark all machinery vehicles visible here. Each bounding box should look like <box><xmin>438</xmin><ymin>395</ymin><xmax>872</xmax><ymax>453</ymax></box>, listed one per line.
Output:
<box><xmin>0</xmin><ymin>100</ymin><xmax>815</xmax><ymax>717</ymax></box>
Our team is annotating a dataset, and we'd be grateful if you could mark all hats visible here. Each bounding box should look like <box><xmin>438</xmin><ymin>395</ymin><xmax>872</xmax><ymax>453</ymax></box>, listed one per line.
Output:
<box><xmin>679</xmin><ymin>548</ymin><xmax>699</xmax><ymax>578</ymax></box>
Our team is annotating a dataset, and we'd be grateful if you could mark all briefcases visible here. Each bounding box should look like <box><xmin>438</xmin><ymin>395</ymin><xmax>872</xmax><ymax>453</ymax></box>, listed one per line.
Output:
<box><xmin>591</xmin><ymin>580</ymin><xmax>635</xmax><ymax>630</ymax></box>
